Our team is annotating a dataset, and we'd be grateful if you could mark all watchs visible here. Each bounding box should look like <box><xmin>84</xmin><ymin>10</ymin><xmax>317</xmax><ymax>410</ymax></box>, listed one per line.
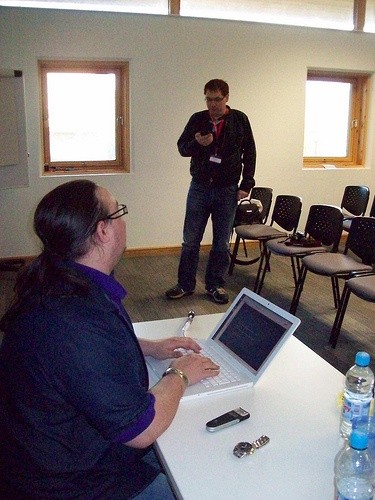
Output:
<box><xmin>233</xmin><ymin>435</ymin><xmax>270</xmax><ymax>458</ymax></box>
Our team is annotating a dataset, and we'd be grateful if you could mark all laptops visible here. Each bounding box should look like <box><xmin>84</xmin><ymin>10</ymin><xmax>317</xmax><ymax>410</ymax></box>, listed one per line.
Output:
<box><xmin>143</xmin><ymin>286</ymin><xmax>303</xmax><ymax>398</ymax></box>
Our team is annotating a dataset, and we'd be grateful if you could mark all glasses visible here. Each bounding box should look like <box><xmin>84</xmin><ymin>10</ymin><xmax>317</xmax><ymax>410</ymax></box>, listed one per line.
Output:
<box><xmin>91</xmin><ymin>204</ymin><xmax>128</xmax><ymax>235</ymax></box>
<box><xmin>204</xmin><ymin>97</ymin><xmax>225</xmax><ymax>102</ymax></box>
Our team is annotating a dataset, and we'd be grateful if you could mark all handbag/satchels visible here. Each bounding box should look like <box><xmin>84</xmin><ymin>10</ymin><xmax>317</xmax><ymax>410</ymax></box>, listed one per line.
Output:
<box><xmin>278</xmin><ymin>233</ymin><xmax>322</xmax><ymax>246</ymax></box>
<box><xmin>237</xmin><ymin>199</ymin><xmax>262</xmax><ymax>224</ymax></box>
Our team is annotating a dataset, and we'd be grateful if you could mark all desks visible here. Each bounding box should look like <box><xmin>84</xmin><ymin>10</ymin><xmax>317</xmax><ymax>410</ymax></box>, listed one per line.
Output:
<box><xmin>131</xmin><ymin>310</ymin><xmax>375</xmax><ymax>500</ymax></box>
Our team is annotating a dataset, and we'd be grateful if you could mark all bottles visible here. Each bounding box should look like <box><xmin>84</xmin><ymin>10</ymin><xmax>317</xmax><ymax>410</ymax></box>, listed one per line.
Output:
<box><xmin>340</xmin><ymin>352</ymin><xmax>375</xmax><ymax>443</ymax></box>
<box><xmin>334</xmin><ymin>428</ymin><xmax>375</xmax><ymax>500</ymax></box>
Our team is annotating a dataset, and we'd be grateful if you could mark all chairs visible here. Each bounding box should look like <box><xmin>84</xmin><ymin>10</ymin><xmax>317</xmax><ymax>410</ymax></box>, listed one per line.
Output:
<box><xmin>225</xmin><ymin>185</ymin><xmax>375</xmax><ymax>349</ymax></box>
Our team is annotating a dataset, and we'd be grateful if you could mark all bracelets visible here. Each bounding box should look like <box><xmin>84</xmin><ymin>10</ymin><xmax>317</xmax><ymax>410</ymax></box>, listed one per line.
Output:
<box><xmin>161</xmin><ymin>367</ymin><xmax>190</xmax><ymax>386</ymax></box>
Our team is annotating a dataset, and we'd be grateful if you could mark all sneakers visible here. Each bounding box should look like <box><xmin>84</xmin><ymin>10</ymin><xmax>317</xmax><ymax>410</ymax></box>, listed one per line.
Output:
<box><xmin>207</xmin><ymin>287</ymin><xmax>229</xmax><ymax>303</ymax></box>
<box><xmin>165</xmin><ymin>284</ymin><xmax>195</xmax><ymax>299</ymax></box>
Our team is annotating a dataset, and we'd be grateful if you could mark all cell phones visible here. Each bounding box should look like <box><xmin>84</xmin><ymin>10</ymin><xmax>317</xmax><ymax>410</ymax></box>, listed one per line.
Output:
<box><xmin>200</xmin><ymin>129</ymin><xmax>210</xmax><ymax>136</ymax></box>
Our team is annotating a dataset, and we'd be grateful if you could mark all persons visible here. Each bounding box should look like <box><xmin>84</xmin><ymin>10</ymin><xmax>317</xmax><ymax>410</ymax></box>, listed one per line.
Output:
<box><xmin>0</xmin><ymin>180</ymin><xmax>221</xmax><ymax>500</ymax></box>
<box><xmin>165</xmin><ymin>79</ymin><xmax>256</xmax><ymax>304</ymax></box>
